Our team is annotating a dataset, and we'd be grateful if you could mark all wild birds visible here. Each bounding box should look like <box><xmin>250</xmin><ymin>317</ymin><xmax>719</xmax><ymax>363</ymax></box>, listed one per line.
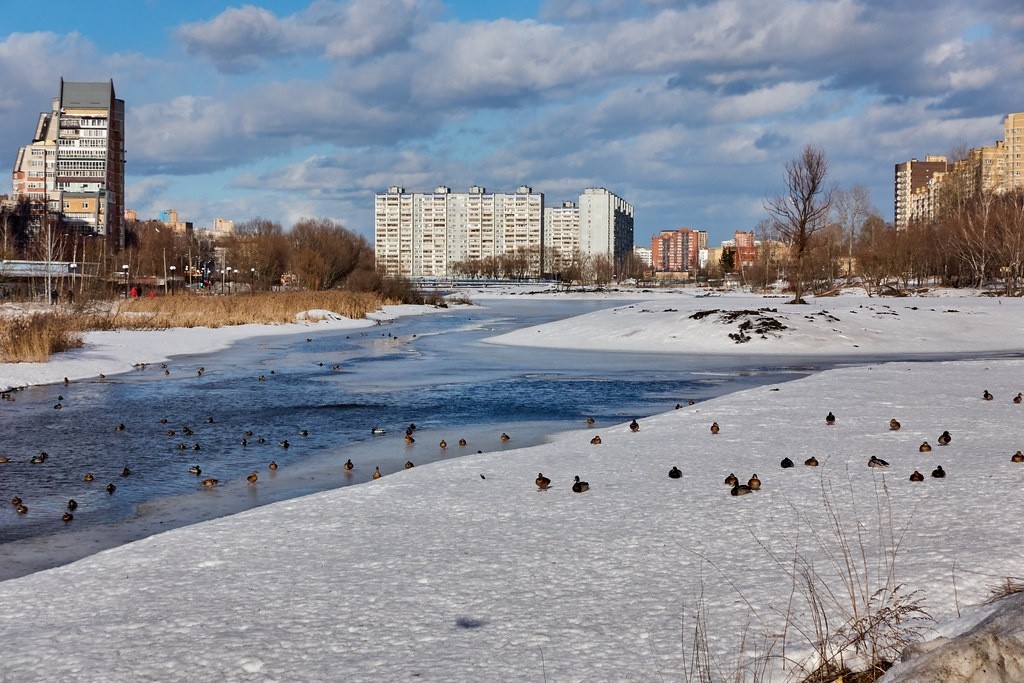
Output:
<box><xmin>919</xmin><ymin>442</ymin><xmax>931</xmax><ymax>452</ymax></box>
<box><xmin>826</xmin><ymin>412</ymin><xmax>835</xmax><ymax>425</ymax></box>
<box><xmin>725</xmin><ymin>473</ymin><xmax>761</xmax><ymax>496</ymax></box>
<box><xmin>937</xmin><ymin>431</ymin><xmax>952</xmax><ymax>445</ymax></box>
<box><xmin>889</xmin><ymin>419</ymin><xmax>900</xmax><ymax>431</ymax></box>
<box><xmin>535</xmin><ymin>473</ymin><xmax>590</xmax><ymax>493</ymax></box>
<box><xmin>983</xmin><ymin>390</ymin><xmax>993</xmax><ymax>400</ymax></box>
<box><xmin>1014</xmin><ymin>393</ymin><xmax>1023</xmax><ymax>403</ymax></box>
<box><xmin>780</xmin><ymin>457</ymin><xmax>794</xmax><ymax>468</ymax></box>
<box><xmin>805</xmin><ymin>456</ymin><xmax>818</xmax><ymax>466</ymax></box>
<box><xmin>133</xmin><ymin>362</ymin><xmax>341</xmax><ymax>382</ymax></box>
<box><xmin>306</xmin><ymin>338</ymin><xmax>312</xmax><ymax>342</ymax></box>
<box><xmin>1012</xmin><ymin>451</ymin><xmax>1024</xmax><ymax>462</ymax></box>
<box><xmin>0</xmin><ymin>417</ymin><xmax>219</xmax><ymax>522</ymax></box>
<box><xmin>868</xmin><ymin>456</ymin><xmax>889</xmax><ymax>468</ymax></box>
<box><xmin>346</xmin><ymin>320</ymin><xmax>416</xmax><ymax>340</ymax></box>
<box><xmin>240</xmin><ymin>416</ymin><xmax>510</xmax><ymax>483</ymax></box>
<box><xmin>932</xmin><ymin>465</ymin><xmax>946</xmax><ymax>478</ymax></box>
<box><xmin>1</xmin><ymin>373</ymin><xmax>104</xmax><ymax>409</ymax></box>
<box><xmin>668</xmin><ymin>466</ymin><xmax>682</xmax><ymax>478</ymax></box>
<box><xmin>587</xmin><ymin>399</ymin><xmax>719</xmax><ymax>444</ymax></box>
<box><xmin>910</xmin><ymin>470</ymin><xmax>924</xmax><ymax>481</ymax></box>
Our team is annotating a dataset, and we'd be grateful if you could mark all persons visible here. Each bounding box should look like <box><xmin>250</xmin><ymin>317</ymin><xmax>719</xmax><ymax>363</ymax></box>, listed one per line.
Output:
<box><xmin>137</xmin><ymin>282</ymin><xmax>143</xmax><ymax>297</ymax></box>
<box><xmin>51</xmin><ymin>288</ymin><xmax>58</xmax><ymax>305</ymax></box>
<box><xmin>150</xmin><ymin>290</ymin><xmax>155</xmax><ymax>298</ymax></box>
<box><xmin>199</xmin><ymin>278</ymin><xmax>216</xmax><ymax>291</ymax></box>
<box><xmin>131</xmin><ymin>287</ymin><xmax>137</xmax><ymax>298</ymax></box>
<box><xmin>66</xmin><ymin>286</ymin><xmax>74</xmax><ymax>304</ymax></box>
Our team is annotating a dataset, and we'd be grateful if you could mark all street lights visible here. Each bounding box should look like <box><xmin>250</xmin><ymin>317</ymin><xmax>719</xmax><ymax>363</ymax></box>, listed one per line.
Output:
<box><xmin>60</xmin><ymin>234</ymin><xmax>68</xmax><ymax>262</ymax></box>
<box><xmin>80</xmin><ymin>234</ymin><xmax>93</xmax><ymax>274</ymax></box>
<box><xmin>234</xmin><ymin>269</ymin><xmax>238</xmax><ymax>293</ymax></box>
<box><xmin>226</xmin><ymin>266</ymin><xmax>231</xmax><ymax>294</ymax></box>
<box><xmin>121</xmin><ymin>264</ymin><xmax>128</xmax><ymax>299</ymax></box>
<box><xmin>251</xmin><ymin>268</ymin><xmax>254</xmax><ymax>286</ymax></box>
<box><xmin>170</xmin><ymin>265</ymin><xmax>176</xmax><ymax>296</ymax></box>
<box><xmin>185</xmin><ymin>266</ymin><xmax>188</xmax><ymax>284</ymax></box>
<box><xmin>221</xmin><ymin>270</ymin><xmax>224</xmax><ymax>294</ymax></box>
<box><xmin>70</xmin><ymin>264</ymin><xmax>77</xmax><ymax>294</ymax></box>
<box><xmin>197</xmin><ymin>268</ymin><xmax>219</xmax><ymax>288</ymax></box>
<box><xmin>36</xmin><ymin>225</ymin><xmax>51</xmax><ymax>305</ymax></box>
